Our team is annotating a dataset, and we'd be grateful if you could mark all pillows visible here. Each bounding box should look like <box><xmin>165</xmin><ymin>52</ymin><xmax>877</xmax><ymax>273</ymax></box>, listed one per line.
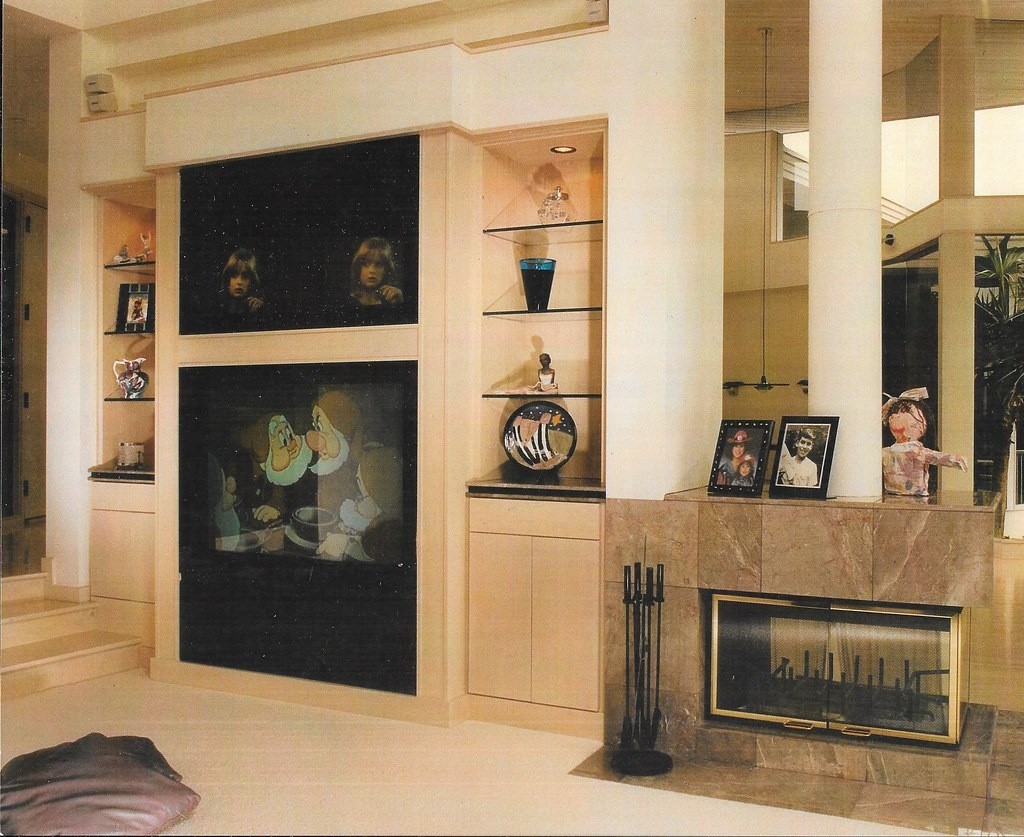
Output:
<box><xmin>0</xmin><ymin>732</ymin><xmax>201</xmax><ymax>836</ymax></box>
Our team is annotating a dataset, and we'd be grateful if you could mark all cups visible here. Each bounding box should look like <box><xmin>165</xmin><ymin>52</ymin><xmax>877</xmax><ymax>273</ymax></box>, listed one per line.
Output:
<box><xmin>520</xmin><ymin>256</ymin><xmax>556</xmax><ymax>311</ymax></box>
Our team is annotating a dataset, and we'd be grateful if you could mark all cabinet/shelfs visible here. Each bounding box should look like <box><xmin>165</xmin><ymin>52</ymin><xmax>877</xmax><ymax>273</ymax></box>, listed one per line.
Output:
<box><xmin>466</xmin><ymin>496</ymin><xmax>602</xmax><ymax>712</ymax></box>
<box><xmin>465</xmin><ymin>219</ymin><xmax>605</xmax><ymax>499</ymax></box>
<box><xmin>91</xmin><ymin>261</ymin><xmax>156</xmax><ymax>482</ymax></box>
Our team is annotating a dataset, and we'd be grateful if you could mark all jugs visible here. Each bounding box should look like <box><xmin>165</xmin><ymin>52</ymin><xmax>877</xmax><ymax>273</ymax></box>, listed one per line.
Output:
<box><xmin>113</xmin><ymin>357</ymin><xmax>149</xmax><ymax>398</ymax></box>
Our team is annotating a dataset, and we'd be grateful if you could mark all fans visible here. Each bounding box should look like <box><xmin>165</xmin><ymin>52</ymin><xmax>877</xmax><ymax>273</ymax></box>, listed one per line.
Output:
<box><xmin>722</xmin><ymin>27</ymin><xmax>790</xmax><ymax>393</ymax></box>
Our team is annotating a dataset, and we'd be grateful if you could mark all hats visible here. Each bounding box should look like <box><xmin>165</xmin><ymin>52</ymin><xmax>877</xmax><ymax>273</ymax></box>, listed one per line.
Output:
<box><xmin>739</xmin><ymin>453</ymin><xmax>754</xmax><ymax>465</ymax></box>
<box><xmin>727</xmin><ymin>431</ymin><xmax>752</xmax><ymax>443</ymax></box>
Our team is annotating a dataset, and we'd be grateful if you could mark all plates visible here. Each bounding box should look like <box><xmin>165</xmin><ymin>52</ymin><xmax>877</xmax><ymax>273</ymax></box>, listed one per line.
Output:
<box><xmin>504</xmin><ymin>401</ymin><xmax>576</xmax><ymax>470</ymax></box>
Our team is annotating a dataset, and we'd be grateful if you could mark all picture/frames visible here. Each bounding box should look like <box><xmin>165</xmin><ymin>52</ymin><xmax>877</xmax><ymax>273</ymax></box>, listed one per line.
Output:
<box><xmin>707</xmin><ymin>419</ymin><xmax>776</xmax><ymax>497</ymax></box>
<box><xmin>769</xmin><ymin>415</ymin><xmax>840</xmax><ymax>500</ymax></box>
<box><xmin>116</xmin><ymin>283</ymin><xmax>155</xmax><ymax>333</ymax></box>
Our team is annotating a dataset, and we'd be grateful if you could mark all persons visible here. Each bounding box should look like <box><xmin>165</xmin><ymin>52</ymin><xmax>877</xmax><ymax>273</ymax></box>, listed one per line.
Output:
<box><xmin>140</xmin><ymin>231</ymin><xmax>151</xmax><ymax>259</ymax></box>
<box><xmin>716</xmin><ymin>430</ymin><xmax>756</xmax><ymax>486</ymax></box>
<box><xmin>124</xmin><ymin>358</ymin><xmax>140</xmax><ymax>386</ymax></box>
<box><xmin>216</xmin><ymin>250</ymin><xmax>266</xmax><ymax>324</ymax></box>
<box><xmin>781</xmin><ymin>429</ymin><xmax>818</xmax><ymax>486</ymax></box>
<box><xmin>119</xmin><ymin>244</ymin><xmax>128</xmax><ymax>259</ymax></box>
<box><xmin>131</xmin><ymin>297</ymin><xmax>143</xmax><ymax>320</ymax></box>
<box><xmin>343</xmin><ymin>237</ymin><xmax>405</xmax><ymax>326</ymax></box>
<box><xmin>538</xmin><ymin>353</ymin><xmax>555</xmax><ymax>390</ymax></box>
